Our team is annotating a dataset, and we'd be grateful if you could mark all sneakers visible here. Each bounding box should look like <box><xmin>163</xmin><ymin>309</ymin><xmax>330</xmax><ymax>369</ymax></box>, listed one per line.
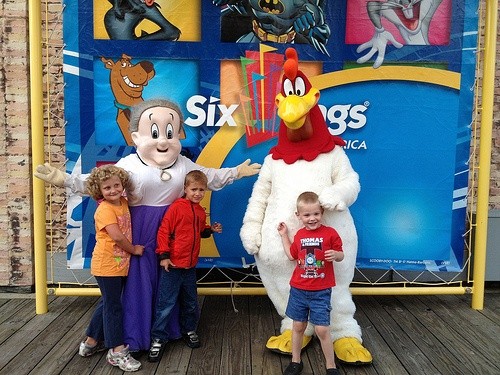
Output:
<box><xmin>78</xmin><ymin>337</ymin><xmax>108</xmax><ymax>357</ymax></box>
<box><xmin>148</xmin><ymin>339</ymin><xmax>165</xmax><ymax>363</ymax></box>
<box><xmin>182</xmin><ymin>331</ymin><xmax>201</xmax><ymax>348</ymax></box>
<box><xmin>106</xmin><ymin>344</ymin><xmax>142</xmax><ymax>372</ymax></box>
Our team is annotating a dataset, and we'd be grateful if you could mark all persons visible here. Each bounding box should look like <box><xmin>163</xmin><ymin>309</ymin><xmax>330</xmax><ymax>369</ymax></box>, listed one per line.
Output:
<box><xmin>277</xmin><ymin>192</ymin><xmax>345</xmax><ymax>375</ymax></box>
<box><xmin>78</xmin><ymin>164</ymin><xmax>144</xmax><ymax>374</ymax></box>
<box><xmin>146</xmin><ymin>170</ymin><xmax>223</xmax><ymax>363</ymax></box>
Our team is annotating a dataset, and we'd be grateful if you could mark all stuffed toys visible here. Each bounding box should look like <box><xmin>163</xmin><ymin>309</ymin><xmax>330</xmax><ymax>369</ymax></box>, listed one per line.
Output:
<box><xmin>34</xmin><ymin>99</ymin><xmax>263</xmax><ymax>354</ymax></box>
<box><xmin>240</xmin><ymin>48</ymin><xmax>375</xmax><ymax>368</ymax></box>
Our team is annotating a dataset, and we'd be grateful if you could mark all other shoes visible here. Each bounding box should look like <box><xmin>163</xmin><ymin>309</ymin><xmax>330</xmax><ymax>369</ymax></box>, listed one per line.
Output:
<box><xmin>283</xmin><ymin>359</ymin><xmax>303</xmax><ymax>375</ymax></box>
<box><xmin>326</xmin><ymin>368</ymin><xmax>340</xmax><ymax>375</ymax></box>
